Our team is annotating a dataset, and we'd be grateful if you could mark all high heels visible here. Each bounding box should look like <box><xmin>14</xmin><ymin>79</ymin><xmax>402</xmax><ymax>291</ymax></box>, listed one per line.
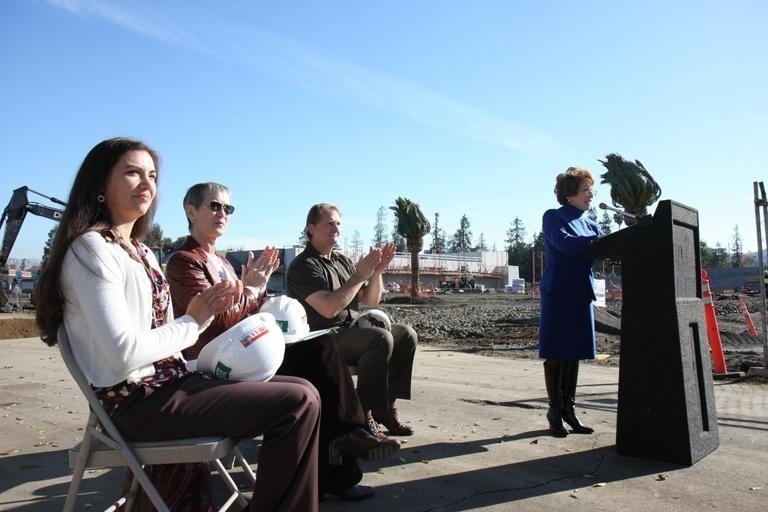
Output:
<box><xmin>318</xmin><ymin>423</ymin><xmax>401</xmax><ymax>503</ymax></box>
<box><xmin>377</xmin><ymin>406</ymin><xmax>414</xmax><ymax>437</ymax></box>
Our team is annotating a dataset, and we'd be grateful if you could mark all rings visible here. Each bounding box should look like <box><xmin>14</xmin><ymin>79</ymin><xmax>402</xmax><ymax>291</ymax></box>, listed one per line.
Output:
<box><xmin>259</xmin><ymin>263</ymin><xmax>264</xmax><ymax>268</ymax></box>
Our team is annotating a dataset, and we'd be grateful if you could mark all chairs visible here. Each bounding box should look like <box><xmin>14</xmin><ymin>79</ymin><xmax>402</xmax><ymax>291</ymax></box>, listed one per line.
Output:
<box><xmin>56</xmin><ymin>322</ymin><xmax>257</xmax><ymax>512</ymax></box>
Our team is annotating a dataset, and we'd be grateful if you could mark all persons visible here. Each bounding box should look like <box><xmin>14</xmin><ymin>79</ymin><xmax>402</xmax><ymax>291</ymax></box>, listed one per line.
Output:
<box><xmin>285</xmin><ymin>203</ymin><xmax>419</xmax><ymax>443</ymax></box>
<box><xmin>538</xmin><ymin>165</ymin><xmax>611</xmax><ymax>437</ymax></box>
<box><xmin>162</xmin><ymin>180</ymin><xmax>404</xmax><ymax>502</ymax></box>
<box><xmin>29</xmin><ymin>137</ymin><xmax>322</xmax><ymax>512</ymax></box>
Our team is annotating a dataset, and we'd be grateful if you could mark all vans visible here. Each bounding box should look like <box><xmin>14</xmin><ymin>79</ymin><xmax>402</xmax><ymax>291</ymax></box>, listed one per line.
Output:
<box><xmin>443</xmin><ymin>274</ymin><xmax>475</xmax><ymax>288</ymax></box>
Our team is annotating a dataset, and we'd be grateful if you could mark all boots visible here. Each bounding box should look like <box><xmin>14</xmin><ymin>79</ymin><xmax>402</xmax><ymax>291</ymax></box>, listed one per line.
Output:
<box><xmin>545</xmin><ymin>376</ymin><xmax>595</xmax><ymax>435</ymax></box>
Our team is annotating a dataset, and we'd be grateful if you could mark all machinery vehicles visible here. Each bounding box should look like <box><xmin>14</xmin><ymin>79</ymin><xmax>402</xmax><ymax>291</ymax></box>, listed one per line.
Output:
<box><xmin>0</xmin><ymin>187</ymin><xmax>68</xmax><ymax>313</ymax></box>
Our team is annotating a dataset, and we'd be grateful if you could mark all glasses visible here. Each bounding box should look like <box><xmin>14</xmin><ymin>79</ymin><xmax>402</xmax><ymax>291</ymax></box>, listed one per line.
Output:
<box><xmin>198</xmin><ymin>201</ymin><xmax>236</xmax><ymax>215</ymax></box>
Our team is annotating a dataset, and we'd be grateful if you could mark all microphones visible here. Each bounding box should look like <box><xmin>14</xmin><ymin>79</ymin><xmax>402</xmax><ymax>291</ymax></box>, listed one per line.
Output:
<box><xmin>599</xmin><ymin>202</ymin><xmax>640</xmax><ymax>221</ymax></box>
